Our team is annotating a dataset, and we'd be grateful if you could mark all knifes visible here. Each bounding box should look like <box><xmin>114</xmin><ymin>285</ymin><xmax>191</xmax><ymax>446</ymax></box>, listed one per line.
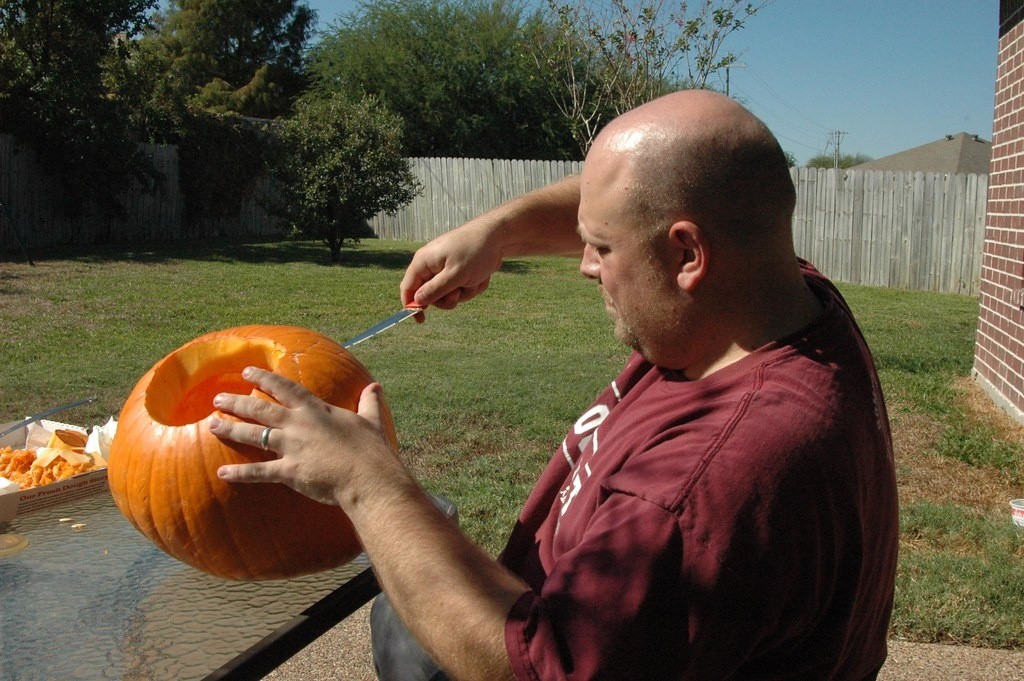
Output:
<box><xmin>341</xmin><ymin>300</ymin><xmax>427</xmax><ymax>350</ymax></box>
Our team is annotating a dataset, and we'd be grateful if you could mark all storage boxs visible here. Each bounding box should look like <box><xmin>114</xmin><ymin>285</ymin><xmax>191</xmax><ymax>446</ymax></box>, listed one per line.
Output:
<box><xmin>0</xmin><ymin>417</ymin><xmax>108</xmax><ymax>519</ymax></box>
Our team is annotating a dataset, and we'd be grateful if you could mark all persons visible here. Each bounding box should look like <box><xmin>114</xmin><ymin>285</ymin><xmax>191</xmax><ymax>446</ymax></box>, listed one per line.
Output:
<box><xmin>208</xmin><ymin>89</ymin><xmax>898</xmax><ymax>681</ymax></box>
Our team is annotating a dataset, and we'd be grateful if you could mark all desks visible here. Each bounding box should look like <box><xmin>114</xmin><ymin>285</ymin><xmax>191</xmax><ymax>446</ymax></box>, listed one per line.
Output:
<box><xmin>0</xmin><ymin>492</ymin><xmax>459</xmax><ymax>681</ymax></box>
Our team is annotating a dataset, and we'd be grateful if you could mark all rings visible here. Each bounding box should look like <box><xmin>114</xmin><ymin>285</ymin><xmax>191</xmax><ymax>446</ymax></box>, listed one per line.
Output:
<box><xmin>261</xmin><ymin>426</ymin><xmax>273</xmax><ymax>451</ymax></box>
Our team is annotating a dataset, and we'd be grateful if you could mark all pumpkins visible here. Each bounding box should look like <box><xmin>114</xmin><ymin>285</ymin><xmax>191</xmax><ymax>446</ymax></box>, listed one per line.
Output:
<box><xmin>108</xmin><ymin>325</ymin><xmax>397</xmax><ymax>580</ymax></box>
